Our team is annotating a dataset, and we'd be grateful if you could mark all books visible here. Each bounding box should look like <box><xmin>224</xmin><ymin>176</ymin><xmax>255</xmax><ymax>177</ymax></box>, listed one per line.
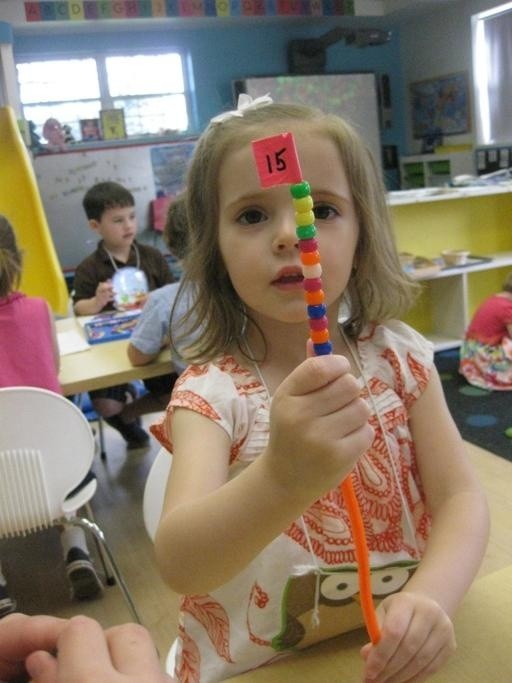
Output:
<box><xmin>79</xmin><ymin>108</ymin><xmax>128</xmax><ymax>141</ymax></box>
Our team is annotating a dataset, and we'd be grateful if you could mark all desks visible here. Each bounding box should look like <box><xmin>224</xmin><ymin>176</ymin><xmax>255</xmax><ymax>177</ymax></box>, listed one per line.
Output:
<box><xmin>221</xmin><ymin>563</ymin><xmax>511</xmax><ymax>677</ymax></box>
<box><xmin>56</xmin><ymin>309</ymin><xmax>176</xmax><ymax>397</ymax></box>
<box><xmin>463</xmin><ymin>442</ymin><xmax>511</xmax><ymax>564</ymax></box>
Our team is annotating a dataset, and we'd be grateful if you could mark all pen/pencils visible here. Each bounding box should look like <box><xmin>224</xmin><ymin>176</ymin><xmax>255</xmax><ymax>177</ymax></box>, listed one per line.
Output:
<box><xmin>87</xmin><ymin>316</ymin><xmax>140</xmax><ymax>338</ymax></box>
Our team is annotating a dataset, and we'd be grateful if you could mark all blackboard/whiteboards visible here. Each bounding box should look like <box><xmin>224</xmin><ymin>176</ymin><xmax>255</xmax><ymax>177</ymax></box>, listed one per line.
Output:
<box><xmin>32</xmin><ymin>137</ymin><xmax>199</xmax><ymax>272</ymax></box>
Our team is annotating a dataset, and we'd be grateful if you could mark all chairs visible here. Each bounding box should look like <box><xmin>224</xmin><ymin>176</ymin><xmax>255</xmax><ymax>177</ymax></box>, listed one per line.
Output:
<box><xmin>1</xmin><ymin>386</ymin><xmax>140</xmax><ymax>624</ymax></box>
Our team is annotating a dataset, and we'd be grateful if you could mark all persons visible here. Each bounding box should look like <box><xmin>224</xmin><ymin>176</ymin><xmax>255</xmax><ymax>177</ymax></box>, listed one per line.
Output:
<box><xmin>126</xmin><ymin>195</ymin><xmax>209</xmax><ymax>375</ymax></box>
<box><xmin>0</xmin><ymin>613</ymin><xmax>167</xmax><ymax>683</ymax></box>
<box><xmin>150</xmin><ymin>95</ymin><xmax>491</xmax><ymax>683</ymax></box>
<box><xmin>0</xmin><ymin>215</ymin><xmax>104</xmax><ymax>615</ymax></box>
<box><xmin>73</xmin><ymin>182</ymin><xmax>177</xmax><ymax>449</ymax></box>
<box><xmin>459</xmin><ymin>276</ymin><xmax>512</xmax><ymax>390</ymax></box>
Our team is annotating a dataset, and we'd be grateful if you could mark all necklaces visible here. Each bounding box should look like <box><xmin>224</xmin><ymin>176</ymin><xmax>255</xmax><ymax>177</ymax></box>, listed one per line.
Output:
<box><xmin>101</xmin><ymin>242</ymin><xmax>141</xmax><ymax>272</ymax></box>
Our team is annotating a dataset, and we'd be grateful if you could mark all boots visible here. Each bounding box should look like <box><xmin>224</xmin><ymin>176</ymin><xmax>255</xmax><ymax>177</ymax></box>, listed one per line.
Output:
<box><xmin>0</xmin><ymin>573</ymin><xmax>17</xmax><ymax>617</ymax></box>
<box><xmin>103</xmin><ymin>406</ymin><xmax>150</xmax><ymax>450</ymax></box>
<box><xmin>61</xmin><ymin>527</ymin><xmax>105</xmax><ymax>600</ymax></box>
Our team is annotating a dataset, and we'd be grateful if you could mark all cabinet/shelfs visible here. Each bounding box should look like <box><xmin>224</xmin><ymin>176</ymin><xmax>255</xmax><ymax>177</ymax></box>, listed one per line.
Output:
<box><xmin>385</xmin><ymin>184</ymin><xmax>511</xmax><ymax>353</ymax></box>
<box><xmin>397</xmin><ymin>149</ymin><xmax>476</xmax><ymax>190</ymax></box>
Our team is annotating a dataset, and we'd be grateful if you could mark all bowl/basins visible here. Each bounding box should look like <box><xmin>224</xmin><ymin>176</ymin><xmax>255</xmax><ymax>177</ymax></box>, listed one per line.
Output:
<box><xmin>441</xmin><ymin>249</ymin><xmax>470</xmax><ymax>266</ymax></box>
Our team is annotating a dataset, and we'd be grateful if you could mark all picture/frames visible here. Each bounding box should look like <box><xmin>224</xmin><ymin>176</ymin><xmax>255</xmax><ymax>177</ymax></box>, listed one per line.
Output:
<box><xmin>407</xmin><ymin>69</ymin><xmax>471</xmax><ymax>138</ymax></box>
